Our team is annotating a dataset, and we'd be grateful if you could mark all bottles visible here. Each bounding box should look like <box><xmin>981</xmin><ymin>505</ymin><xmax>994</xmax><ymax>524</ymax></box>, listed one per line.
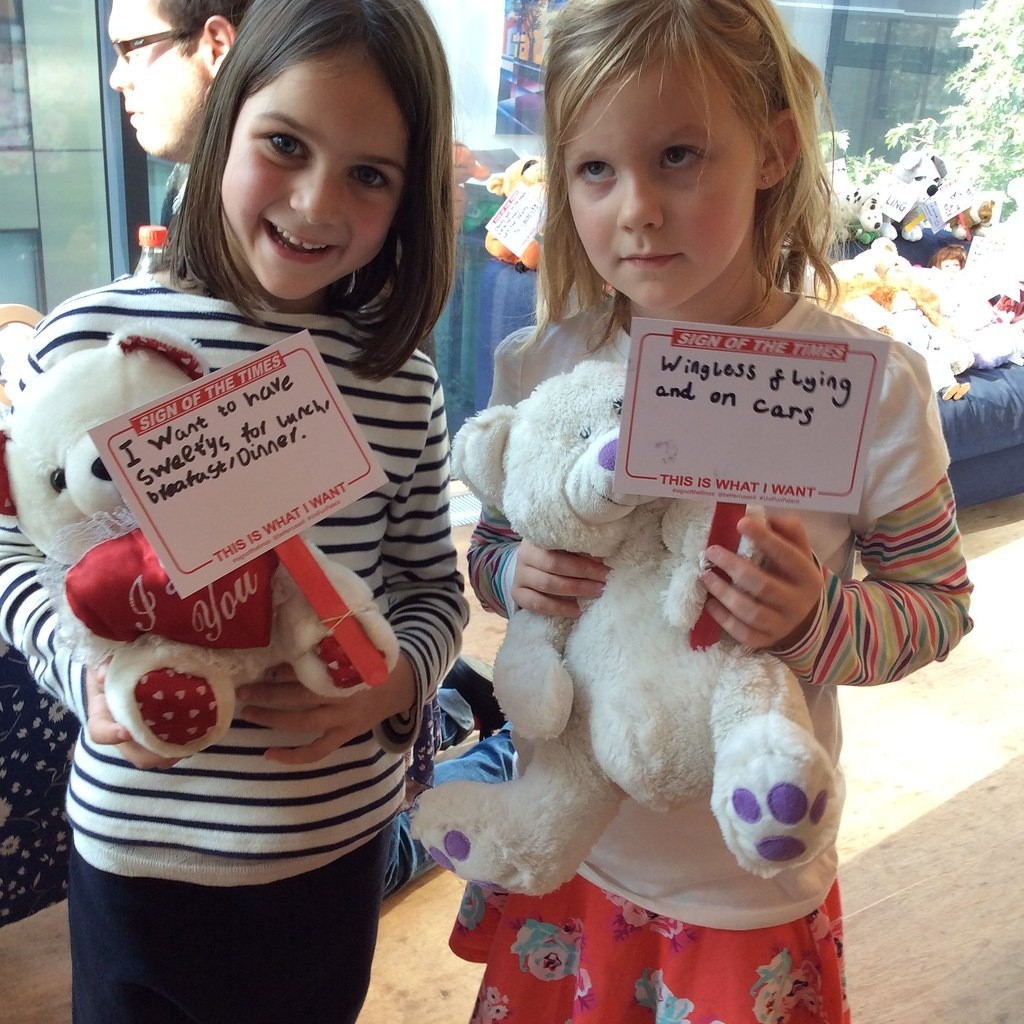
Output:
<box><xmin>130</xmin><ymin>225</ymin><xmax>174</xmax><ymax>276</ymax></box>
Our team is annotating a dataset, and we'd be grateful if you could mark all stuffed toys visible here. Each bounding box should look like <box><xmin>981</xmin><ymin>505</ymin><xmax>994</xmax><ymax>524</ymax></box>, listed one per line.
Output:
<box><xmin>0</xmin><ymin>316</ymin><xmax>400</xmax><ymax>759</ymax></box>
<box><xmin>407</xmin><ymin>360</ymin><xmax>847</xmax><ymax>896</ymax></box>
<box><xmin>485</xmin><ymin>157</ymin><xmax>544</xmax><ymax>273</ymax></box>
<box><xmin>812</xmin><ymin>151</ymin><xmax>1024</xmax><ymax>400</ymax></box>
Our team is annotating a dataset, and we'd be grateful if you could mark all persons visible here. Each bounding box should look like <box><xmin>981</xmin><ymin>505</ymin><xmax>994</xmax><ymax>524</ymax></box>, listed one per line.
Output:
<box><xmin>0</xmin><ymin>0</ymin><xmax>470</xmax><ymax>1024</ymax></box>
<box><xmin>449</xmin><ymin>0</ymin><xmax>974</xmax><ymax>1024</ymax></box>
<box><xmin>108</xmin><ymin>0</ymin><xmax>514</xmax><ymax>901</ymax></box>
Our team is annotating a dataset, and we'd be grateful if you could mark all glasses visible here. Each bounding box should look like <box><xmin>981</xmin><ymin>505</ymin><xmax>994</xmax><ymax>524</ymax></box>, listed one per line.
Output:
<box><xmin>111</xmin><ymin>15</ymin><xmax>247</xmax><ymax>64</ymax></box>
